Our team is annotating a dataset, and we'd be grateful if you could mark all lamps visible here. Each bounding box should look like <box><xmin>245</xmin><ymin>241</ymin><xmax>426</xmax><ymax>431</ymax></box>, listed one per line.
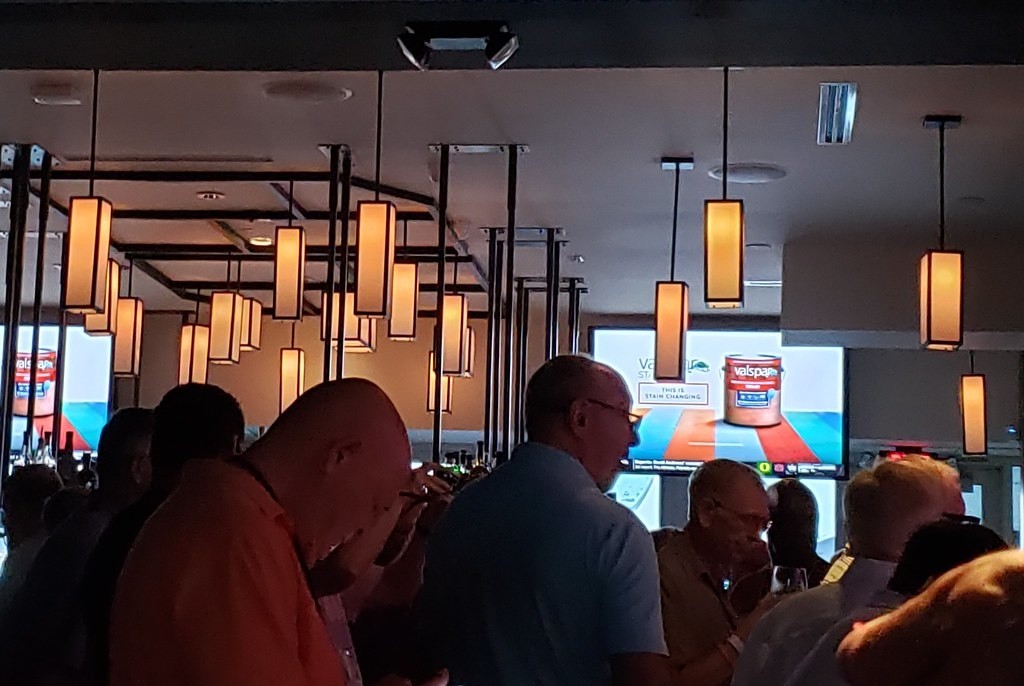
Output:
<box><xmin>64</xmin><ymin>69</ymin><xmax>113</xmax><ymax>314</ymax></box>
<box><xmin>653</xmin><ymin>155</ymin><xmax>694</xmax><ymax>382</ymax></box>
<box><xmin>85</xmin><ymin>258</ymin><xmax>121</xmax><ymax>336</ymax></box>
<box><xmin>177</xmin><ymin>72</ymin><xmax>477</xmax><ymax>415</ymax></box>
<box><xmin>397</xmin><ymin>20</ymin><xmax>521</xmax><ymax>71</ymax></box>
<box><xmin>918</xmin><ymin>115</ymin><xmax>965</xmax><ymax>352</ymax></box>
<box><xmin>961</xmin><ymin>350</ymin><xmax>990</xmax><ymax>458</ymax></box>
<box><xmin>110</xmin><ymin>261</ymin><xmax>144</xmax><ymax>378</ymax></box>
<box><xmin>703</xmin><ymin>67</ymin><xmax>745</xmax><ymax>308</ymax></box>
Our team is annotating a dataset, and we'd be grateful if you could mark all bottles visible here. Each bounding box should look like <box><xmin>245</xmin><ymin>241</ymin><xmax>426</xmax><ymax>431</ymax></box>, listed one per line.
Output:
<box><xmin>12</xmin><ymin>429</ymin><xmax>98</xmax><ymax>491</ymax></box>
<box><xmin>439</xmin><ymin>440</ymin><xmax>503</xmax><ymax>480</ymax></box>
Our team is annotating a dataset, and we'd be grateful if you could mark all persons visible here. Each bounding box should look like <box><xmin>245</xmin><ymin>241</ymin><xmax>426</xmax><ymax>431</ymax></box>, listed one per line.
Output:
<box><xmin>110</xmin><ymin>378</ymin><xmax>411</xmax><ymax>686</ymax></box>
<box><xmin>41</xmin><ymin>487</ymin><xmax>96</xmax><ymax>536</ymax></box>
<box><xmin>81</xmin><ymin>383</ymin><xmax>244</xmax><ymax>686</ymax></box>
<box><xmin>0</xmin><ymin>463</ymin><xmax>66</xmax><ymax>607</ymax></box>
<box><xmin>20</xmin><ymin>407</ymin><xmax>157</xmax><ymax>686</ymax></box>
<box><xmin>313</xmin><ymin>355</ymin><xmax>1021</xmax><ymax>686</ymax></box>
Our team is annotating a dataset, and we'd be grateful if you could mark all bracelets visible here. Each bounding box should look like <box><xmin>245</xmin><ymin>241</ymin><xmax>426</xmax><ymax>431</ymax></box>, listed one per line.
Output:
<box><xmin>718</xmin><ymin>645</ymin><xmax>736</xmax><ymax>668</ymax></box>
<box><xmin>727</xmin><ymin>634</ymin><xmax>743</xmax><ymax>653</ymax></box>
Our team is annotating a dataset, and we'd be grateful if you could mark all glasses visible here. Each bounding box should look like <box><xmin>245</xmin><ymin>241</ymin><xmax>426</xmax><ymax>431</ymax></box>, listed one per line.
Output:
<box><xmin>941</xmin><ymin>512</ymin><xmax>980</xmax><ymax>526</ymax></box>
<box><xmin>568</xmin><ymin>395</ymin><xmax>642</xmax><ymax>433</ymax></box>
<box><xmin>708</xmin><ymin>499</ymin><xmax>770</xmax><ymax>533</ymax></box>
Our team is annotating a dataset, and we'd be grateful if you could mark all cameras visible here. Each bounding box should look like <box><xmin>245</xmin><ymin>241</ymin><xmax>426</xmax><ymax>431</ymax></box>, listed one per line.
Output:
<box><xmin>428</xmin><ymin>466</ymin><xmax>459</xmax><ymax>499</ymax></box>
